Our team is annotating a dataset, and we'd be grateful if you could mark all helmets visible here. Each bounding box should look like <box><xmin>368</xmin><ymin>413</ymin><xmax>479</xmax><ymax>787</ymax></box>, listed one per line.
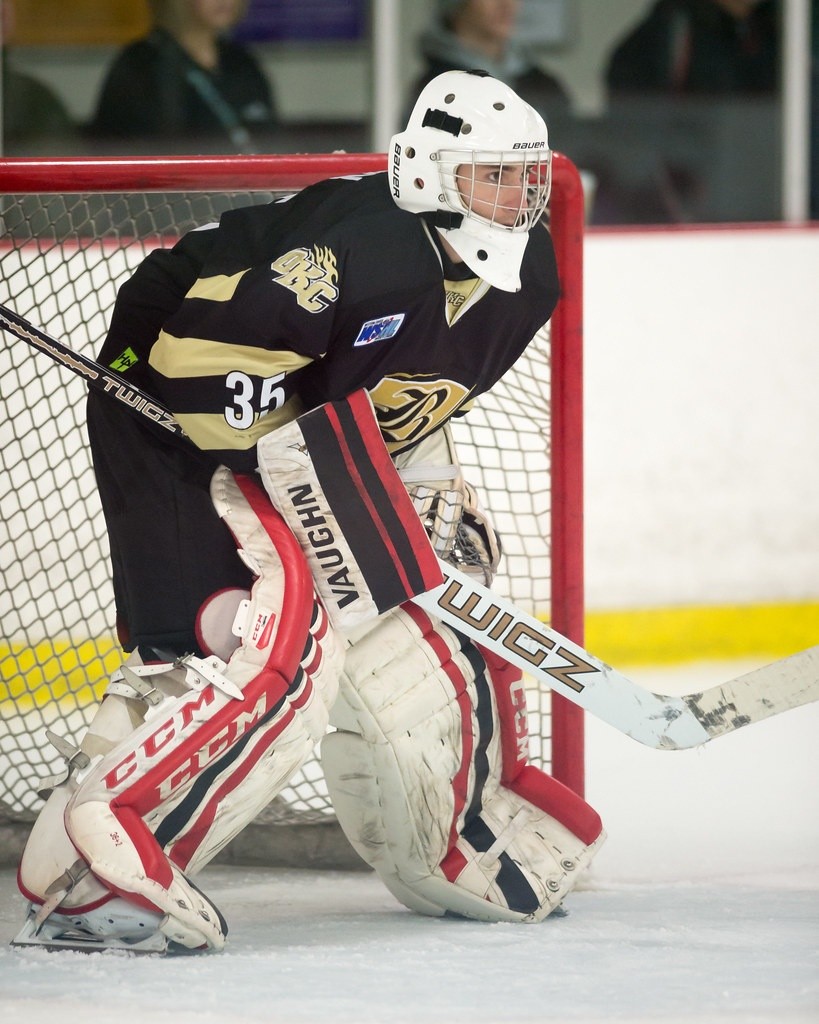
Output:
<box><xmin>387</xmin><ymin>72</ymin><xmax>548</xmax><ymax>293</ymax></box>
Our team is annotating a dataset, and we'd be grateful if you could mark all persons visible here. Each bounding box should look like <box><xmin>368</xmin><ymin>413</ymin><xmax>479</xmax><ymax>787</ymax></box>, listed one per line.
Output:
<box><xmin>0</xmin><ymin>6</ymin><xmax>76</xmax><ymax>157</ymax></box>
<box><xmin>18</xmin><ymin>69</ymin><xmax>604</xmax><ymax>954</ymax></box>
<box><xmin>403</xmin><ymin>0</ymin><xmax>581</xmax><ymax>171</ymax></box>
<box><xmin>589</xmin><ymin>0</ymin><xmax>819</xmax><ymax>224</ymax></box>
<box><xmin>90</xmin><ymin>0</ymin><xmax>283</xmax><ymax>148</ymax></box>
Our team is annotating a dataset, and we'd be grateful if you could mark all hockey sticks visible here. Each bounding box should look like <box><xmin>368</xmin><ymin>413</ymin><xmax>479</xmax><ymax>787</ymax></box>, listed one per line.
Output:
<box><xmin>0</xmin><ymin>305</ymin><xmax>819</xmax><ymax>751</ymax></box>
